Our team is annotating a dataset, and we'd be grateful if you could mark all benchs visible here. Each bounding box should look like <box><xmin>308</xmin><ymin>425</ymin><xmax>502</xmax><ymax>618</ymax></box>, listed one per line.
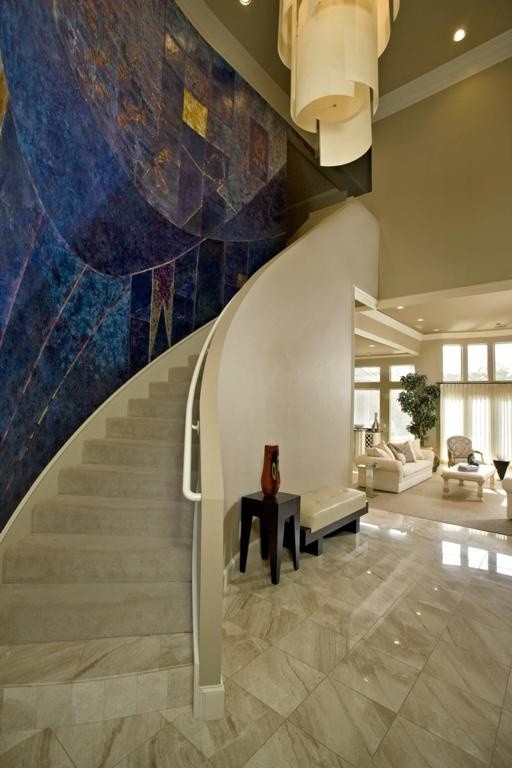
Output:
<box><xmin>283</xmin><ymin>485</ymin><xmax>369</xmax><ymax>556</ymax></box>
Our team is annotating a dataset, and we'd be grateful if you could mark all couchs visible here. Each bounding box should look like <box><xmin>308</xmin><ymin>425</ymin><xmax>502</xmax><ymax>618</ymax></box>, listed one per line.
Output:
<box><xmin>353</xmin><ymin>443</ymin><xmax>435</xmax><ymax>493</ymax></box>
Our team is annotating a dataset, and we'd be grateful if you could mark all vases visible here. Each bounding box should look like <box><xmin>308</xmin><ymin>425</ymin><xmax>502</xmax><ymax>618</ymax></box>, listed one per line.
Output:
<box><xmin>260</xmin><ymin>444</ymin><xmax>280</xmax><ymax>496</ymax></box>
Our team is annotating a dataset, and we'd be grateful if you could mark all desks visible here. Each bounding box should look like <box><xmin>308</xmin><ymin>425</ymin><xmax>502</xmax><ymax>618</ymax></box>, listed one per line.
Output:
<box><xmin>239</xmin><ymin>490</ymin><xmax>301</xmax><ymax>584</ymax></box>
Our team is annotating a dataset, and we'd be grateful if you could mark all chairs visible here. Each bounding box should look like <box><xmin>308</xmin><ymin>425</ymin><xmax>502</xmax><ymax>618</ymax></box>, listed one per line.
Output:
<box><xmin>446</xmin><ymin>436</ymin><xmax>487</xmax><ymax>467</ymax></box>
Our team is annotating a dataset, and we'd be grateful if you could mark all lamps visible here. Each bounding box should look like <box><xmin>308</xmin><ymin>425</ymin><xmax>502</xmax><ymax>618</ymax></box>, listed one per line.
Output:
<box><xmin>276</xmin><ymin>0</ymin><xmax>400</xmax><ymax>168</ymax></box>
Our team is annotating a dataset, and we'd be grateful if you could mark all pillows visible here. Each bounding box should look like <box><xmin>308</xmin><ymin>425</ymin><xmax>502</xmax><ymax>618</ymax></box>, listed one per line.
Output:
<box><xmin>364</xmin><ymin>439</ymin><xmax>424</xmax><ymax>465</ymax></box>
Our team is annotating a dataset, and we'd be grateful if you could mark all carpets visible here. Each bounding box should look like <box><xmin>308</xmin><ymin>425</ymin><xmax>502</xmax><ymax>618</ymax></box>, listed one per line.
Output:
<box><xmin>353</xmin><ymin>469</ymin><xmax>512</xmax><ymax>537</ymax></box>
